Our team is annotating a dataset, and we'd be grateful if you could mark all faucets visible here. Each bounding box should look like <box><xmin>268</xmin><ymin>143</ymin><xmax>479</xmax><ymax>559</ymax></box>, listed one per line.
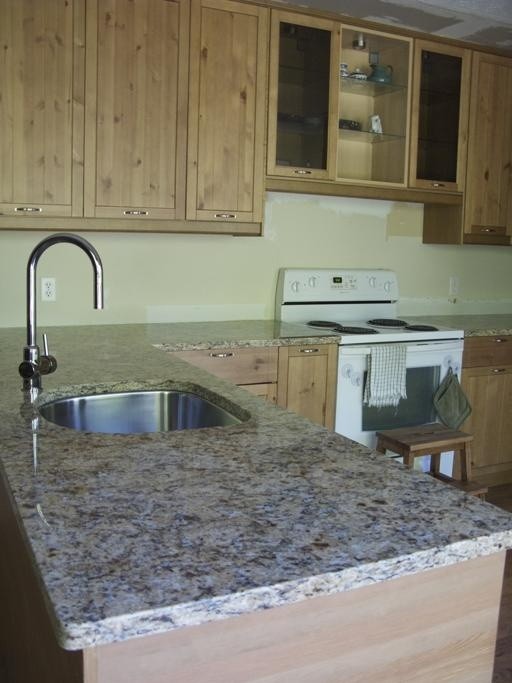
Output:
<box><xmin>18</xmin><ymin>232</ymin><xmax>104</xmax><ymax>390</ymax></box>
<box><xmin>19</xmin><ymin>390</ymin><xmax>54</xmax><ymax>531</ymax></box>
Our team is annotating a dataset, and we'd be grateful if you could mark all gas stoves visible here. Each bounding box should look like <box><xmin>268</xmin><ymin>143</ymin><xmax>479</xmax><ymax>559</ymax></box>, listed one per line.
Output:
<box><xmin>284</xmin><ymin>318</ymin><xmax>463</xmax><ymax>342</ymax></box>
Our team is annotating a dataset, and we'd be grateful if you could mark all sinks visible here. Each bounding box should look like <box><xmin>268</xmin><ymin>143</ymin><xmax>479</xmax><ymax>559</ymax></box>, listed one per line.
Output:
<box><xmin>36</xmin><ymin>378</ymin><xmax>252</xmax><ymax>434</ymax></box>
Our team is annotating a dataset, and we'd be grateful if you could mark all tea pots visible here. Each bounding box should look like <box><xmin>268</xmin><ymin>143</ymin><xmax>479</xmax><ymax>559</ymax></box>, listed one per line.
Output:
<box><xmin>368</xmin><ymin>63</ymin><xmax>395</xmax><ymax>85</ymax></box>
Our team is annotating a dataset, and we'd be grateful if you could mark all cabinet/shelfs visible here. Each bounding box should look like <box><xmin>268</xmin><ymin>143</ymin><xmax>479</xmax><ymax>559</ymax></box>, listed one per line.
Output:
<box><xmin>263</xmin><ymin>0</ymin><xmax>475</xmax><ymax>208</ymax></box>
<box><xmin>461</xmin><ymin>335</ymin><xmax>512</xmax><ymax>490</ymax></box>
<box><xmin>279</xmin><ymin>342</ymin><xmax>339</xmax><ymax>436</ymax></box>
<box><xmin>421</xmin><ymin>30</ymin><xmax>512</xmax><ymax>247</ymax></box>
<box><xmin>165</xmin><ymin>346</ymin><xmax>278</xmax><ymax>404</ymax></box>
<box><xmin>0</xmin><ymin>0</ymin><xmax>271</xmax><ymax>238</ymax></box>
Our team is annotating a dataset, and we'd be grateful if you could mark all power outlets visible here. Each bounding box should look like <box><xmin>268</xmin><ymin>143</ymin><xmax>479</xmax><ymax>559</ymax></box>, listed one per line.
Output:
<box><xmin>40</xmin><ymin>276</ymin><xmax>54</xmax><ymax>301</ymax></box>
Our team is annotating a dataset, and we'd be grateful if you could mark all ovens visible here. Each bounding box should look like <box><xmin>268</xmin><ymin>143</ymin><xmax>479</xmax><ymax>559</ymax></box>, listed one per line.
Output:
<box><xmin>334</xmin><ymin>339</ymin><xmax>465</xmax><ymax>479</ymax></box>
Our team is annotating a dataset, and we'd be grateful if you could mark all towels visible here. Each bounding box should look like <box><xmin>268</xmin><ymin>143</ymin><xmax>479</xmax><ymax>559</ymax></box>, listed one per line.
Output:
<box><xmin>362</xmin><ymin>342</ymin><xmax>408</xmax><ymax>408</ymax></box>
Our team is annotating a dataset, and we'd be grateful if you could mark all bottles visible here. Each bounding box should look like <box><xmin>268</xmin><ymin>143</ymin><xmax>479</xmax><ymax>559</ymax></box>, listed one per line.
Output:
<box><xmin>340</xmin><ymin>63</ymin><xmax>350</xmax><ymax>78</ymax></box>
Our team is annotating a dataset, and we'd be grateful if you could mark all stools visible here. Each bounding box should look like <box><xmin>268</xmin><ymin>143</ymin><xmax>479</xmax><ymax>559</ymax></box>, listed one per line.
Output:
<box><xmin>376</xmin><ymin>422</ymin><xmax>489</xmax><ymax>503</ymax></box>
